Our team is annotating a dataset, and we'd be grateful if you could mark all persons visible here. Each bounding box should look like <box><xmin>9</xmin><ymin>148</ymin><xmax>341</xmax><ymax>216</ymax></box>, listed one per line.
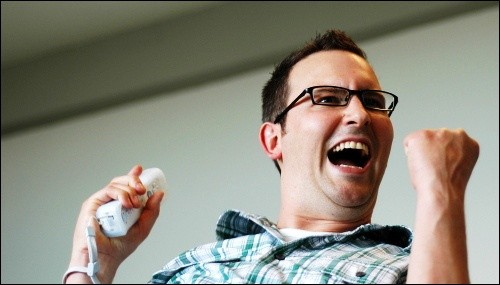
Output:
<box><xmin>60</xmin><ymin>22</ymin><xmax>481</xmax><ymax>284</ymax></box>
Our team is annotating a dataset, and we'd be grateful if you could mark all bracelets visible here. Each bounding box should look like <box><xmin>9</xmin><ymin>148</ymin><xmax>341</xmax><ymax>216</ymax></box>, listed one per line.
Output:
<box><xmin>62</xmin><ymin>267</ymin><xmax>100</xmax><ymax>284</ymax></box>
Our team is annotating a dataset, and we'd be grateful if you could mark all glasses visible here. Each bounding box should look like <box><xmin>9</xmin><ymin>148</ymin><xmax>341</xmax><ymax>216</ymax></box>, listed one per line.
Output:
<box><xmin>271</xmin><ymin>85</ymin><xmax>399</xmax><ymax>126</ymax></box>
